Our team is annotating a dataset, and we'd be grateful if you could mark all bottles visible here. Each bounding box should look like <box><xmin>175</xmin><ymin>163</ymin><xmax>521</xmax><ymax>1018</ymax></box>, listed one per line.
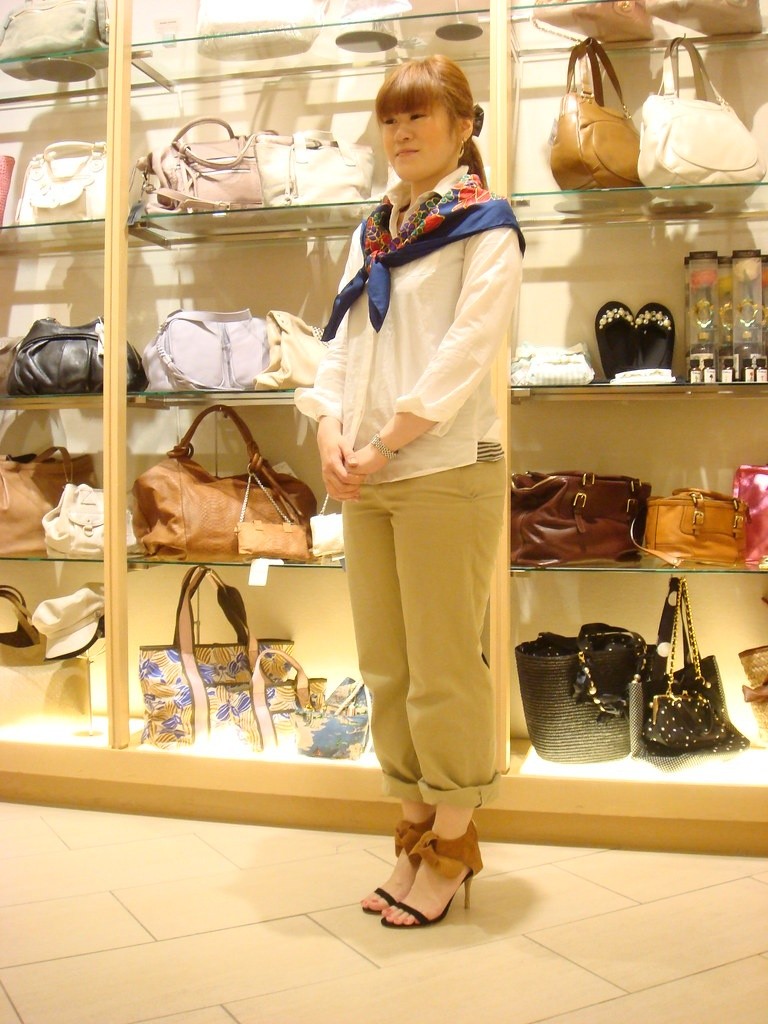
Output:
<box><xmin>688</xmin><ymin>359</ymin><xmax>768</xmax><ymax>384</ymax></box>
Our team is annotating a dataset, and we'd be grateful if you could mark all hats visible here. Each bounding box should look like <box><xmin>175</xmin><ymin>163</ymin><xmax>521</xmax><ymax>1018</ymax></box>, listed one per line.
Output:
<box><xmin>33</xmin><ymin>582</ymin><xmax>104</xmax><ymax>660</ymax></box>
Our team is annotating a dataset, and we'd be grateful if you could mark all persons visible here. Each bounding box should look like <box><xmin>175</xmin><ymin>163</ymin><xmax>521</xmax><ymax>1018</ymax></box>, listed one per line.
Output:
<box><xmin>295</xmin><ymin>54</ymin><xmax>527</xmax><ymax>927</ymax></box>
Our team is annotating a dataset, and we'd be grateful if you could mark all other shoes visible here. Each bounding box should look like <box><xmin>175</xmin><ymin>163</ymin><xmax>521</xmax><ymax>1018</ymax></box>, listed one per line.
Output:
<box><xmin>596</xmin><ymin>300</ymin><xmax>637</xmax><ymax>379</ymax></box>
<box><xmin>635</xmin><ymin>302</ymin><xmax>675</xmax><ymax>369</ymax></box>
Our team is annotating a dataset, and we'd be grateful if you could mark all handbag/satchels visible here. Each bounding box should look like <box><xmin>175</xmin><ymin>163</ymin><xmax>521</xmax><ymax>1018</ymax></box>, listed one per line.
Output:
<box><xmin>515</xmin><ymin>622</ymin><xmax>654</xmax><ymax>763</ymax></box>
<box><xmin>13</xmin><ymin>140</ymin><xmax>105</xmax><ymax>221</ymax></box>
<box><xmin>0</xmin><ymin>585</ymin><xmax>91</xmax><ymax>736</ymax></box>
<box><xmin>732</xmin><ymin>463</ymin><xmax>768</xmax><ymax>564</ymax></box>
<box><xmin>737</xmin><ymin>645</ymin><xmax>767</xmax><ymax>751</ymax></box>
<box><xmin>511</xmin><ymin>470</ymin><xmax>652</xmax><ymax>568</ymax></box>
<box><xmin>139</xmin><ymin>567</ymin><xmax>294</xmax><ymax>743</ymax></box>
<box><xmin>0</xmin><ymin>0</ymin><xmax>111</xmax><ymax>80</ymax></box>
<box><xmin>512</xmin><ymin>344</ymin><xmax>594</xmax><ymax>385</ymax></box>
<box><xmin>0</xmin><ymin>404</ymin><xmax>347</xmax><ymax>562</ymax></box>
<box><xmin>142</xmin><ymin>308</ymin><xmax>269</xmax><ymax>392</ymax></box>
<box><xmin>230</xmin><ymin>647</ymin><xmax>369</xmax><ymax>760</ymax></box>
<box><xmin>130</xmin><ymin>117</ymin><xmax>279</xmax><ymax>212</ymax></box>
<box><xmin>628</xmin><ymin>578</ymin><xmax>751</xmax><ymax>771</ymax></box>
<box><xmin>551</xmin><ymin>38</ymin><xmax>640</xmax><ymax>188</ymax></box>
<box><xmin>255</xmin><ymin>131</ymin><xmax>372</xmax><ymax>208</ymax></box>
<box><xmin>533</xmin><ymin>0</ymin><xmax>765</xmax><ymax>39</ymax></box>
<box><xmin>643</xmin><ymin>488</ymin><xmax>751</xmax><ymax>568</ymax></box>
<box><xmin>636</xmin><ymin>39</ymin><xmax>764</xmax><ymax>187</ymax></box>
<box><xmin>256</xmin><ymin>311</ymin><xmax>326</xmax><ymax>389</ymax></box>
<box><xmin>7</xmin><ymin>317</ymin><xmax>148</xmax><ymax>394</ymax></box>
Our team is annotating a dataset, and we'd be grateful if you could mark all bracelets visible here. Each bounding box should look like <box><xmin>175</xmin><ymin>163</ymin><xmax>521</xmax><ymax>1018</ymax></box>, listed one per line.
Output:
<box><xmin>371</xmin><ymin>434</ymin><xmax>398</xmax><ymax>459</ymax></box>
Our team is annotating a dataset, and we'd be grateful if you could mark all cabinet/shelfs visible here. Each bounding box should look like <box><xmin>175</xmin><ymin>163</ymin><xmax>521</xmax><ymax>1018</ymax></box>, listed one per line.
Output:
<box><xmin>1</xmin><ymin>0</ymin><xmax>768</xmax><ymax>856</ymax></box>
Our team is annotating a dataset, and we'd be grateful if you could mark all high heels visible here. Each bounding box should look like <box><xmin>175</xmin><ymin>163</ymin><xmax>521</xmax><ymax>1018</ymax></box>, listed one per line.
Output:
<box><xmin>381</xmin><ymin>818</ymin><xmax>483</xmax><ymax>928</ymax></box>
<box><xmin>361</xmin><ymin>813</ymin><xmax>435</xmax><ymax>913</ymax></box>
